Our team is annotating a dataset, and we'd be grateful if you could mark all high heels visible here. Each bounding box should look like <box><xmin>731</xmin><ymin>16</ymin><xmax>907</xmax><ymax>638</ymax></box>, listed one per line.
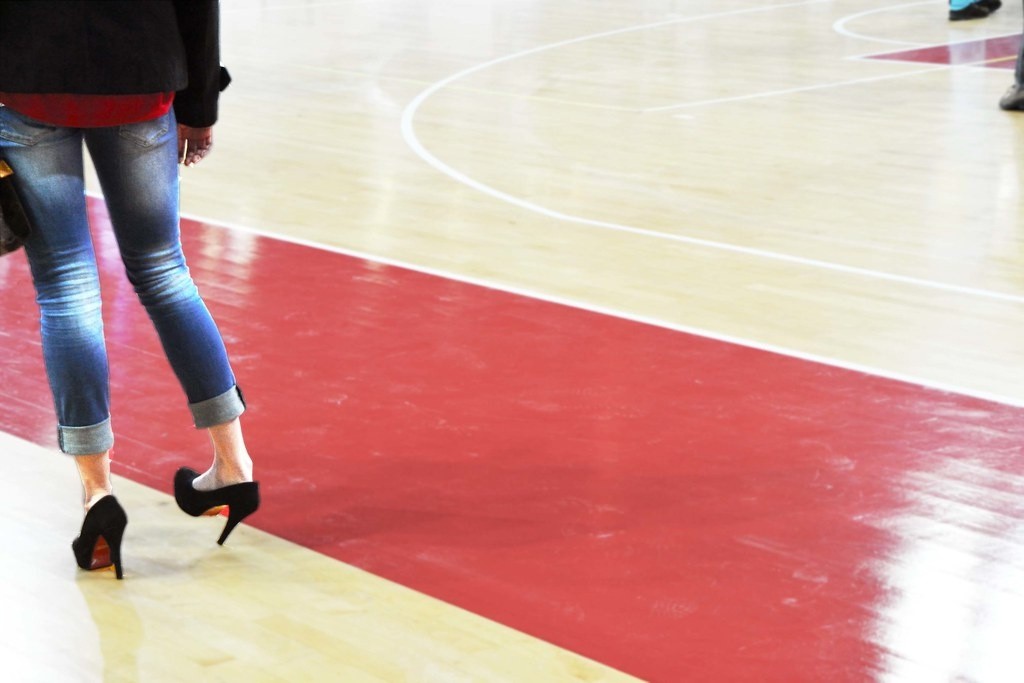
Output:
<box><xmin>72</xmin><ymin>494</ymin><xmax>127</xmax><ymax>580</ymax></box>
<box><xmin>174</xmin><ymin>466</ymin><xmax>261</xmax><ymax>545</ymax></box>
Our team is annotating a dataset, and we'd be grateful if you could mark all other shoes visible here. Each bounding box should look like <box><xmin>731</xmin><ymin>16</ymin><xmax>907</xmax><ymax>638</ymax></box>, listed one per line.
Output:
<box><xmin>949</xmin><ymin>0</ymin><xmax>1001</xmax><ymax>20</ymax></box>
<box><xmin>999</xmin><ymin>83</ymin><xmax>1024</xmax><ymax>108</ymax></box>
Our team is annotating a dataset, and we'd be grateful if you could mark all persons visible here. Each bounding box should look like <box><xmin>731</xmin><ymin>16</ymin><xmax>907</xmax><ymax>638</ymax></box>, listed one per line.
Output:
<box><xmin>0</xmin><ymin>0</ymin><xmax>259</xmax><ymax>580</ymax></box>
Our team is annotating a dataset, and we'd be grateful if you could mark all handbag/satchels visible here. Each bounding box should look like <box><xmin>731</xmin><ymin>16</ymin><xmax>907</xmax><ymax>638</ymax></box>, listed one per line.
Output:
<box><xmin>0</xmin><ymin>161</ymin><xmax>30</xmax><ymax>257</ymax></box>
